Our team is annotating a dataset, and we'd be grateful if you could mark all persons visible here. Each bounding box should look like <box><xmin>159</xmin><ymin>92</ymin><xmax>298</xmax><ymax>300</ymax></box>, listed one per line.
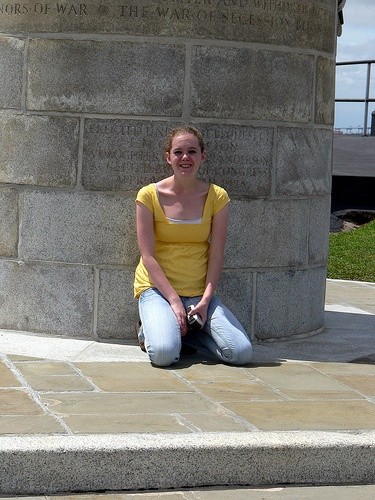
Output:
<box><xmin>134</xmin><ymin>126</ymin><xmax>253</xmax><ymax>367</ymax></box>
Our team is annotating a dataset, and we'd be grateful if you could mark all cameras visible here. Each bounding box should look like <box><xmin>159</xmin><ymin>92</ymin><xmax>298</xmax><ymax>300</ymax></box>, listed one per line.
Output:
<box><xmin>187</xmin><ymin>305</ymin><xmax>204</xmax><ymax>329</ymax></box>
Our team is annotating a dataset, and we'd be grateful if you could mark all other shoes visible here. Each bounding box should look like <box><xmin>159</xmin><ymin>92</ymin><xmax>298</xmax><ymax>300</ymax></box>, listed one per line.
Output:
<box><xmin>135</xmin><ymin>320</ymin><xmax>146</xmax><ymax>352</ymax></box>
